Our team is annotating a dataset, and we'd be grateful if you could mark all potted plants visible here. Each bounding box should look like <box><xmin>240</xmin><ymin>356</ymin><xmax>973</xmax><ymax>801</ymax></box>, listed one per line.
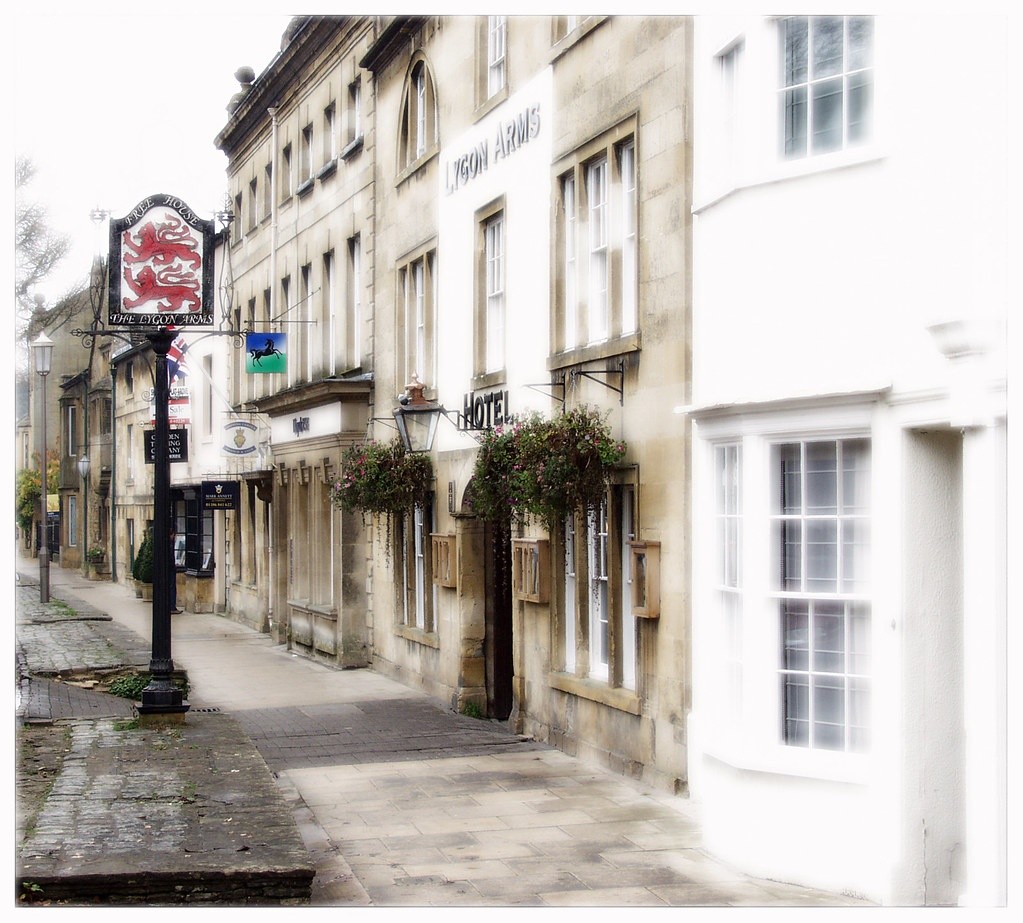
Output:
<box><xmin>131</xmin><ymin>526</ymin><xmax>154</xmax><ymax>602</ymax></box>
<box><xmin>87</xmin><ymin>547</ymin><xmax>107</xmax><ymax>563</ymax></box>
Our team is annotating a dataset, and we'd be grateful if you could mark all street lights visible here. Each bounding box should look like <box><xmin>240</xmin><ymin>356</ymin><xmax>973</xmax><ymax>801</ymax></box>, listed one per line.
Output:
<box><xmin>30</xmin><ymin>331</ymin><xmax>56</xmax><ymax>603</ymax></box>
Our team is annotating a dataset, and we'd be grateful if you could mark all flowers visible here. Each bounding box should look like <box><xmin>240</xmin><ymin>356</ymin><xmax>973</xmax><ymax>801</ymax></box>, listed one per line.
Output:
<box><xmin>321</xmin><ymin>436</ymin><xmax>437</xmax><ymax>564</ymax></box>
<box><xmin>464</xmin><ymin>410</ymin><xmax>629</xmax><ymax>611</ymax></box>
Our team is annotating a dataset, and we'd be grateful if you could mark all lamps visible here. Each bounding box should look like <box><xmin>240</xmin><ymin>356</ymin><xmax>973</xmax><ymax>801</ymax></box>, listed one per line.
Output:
<box><xmin>397</xmin><ymin>372</ymin><xmax>495</xmax><ymax>455</ymax></box>
